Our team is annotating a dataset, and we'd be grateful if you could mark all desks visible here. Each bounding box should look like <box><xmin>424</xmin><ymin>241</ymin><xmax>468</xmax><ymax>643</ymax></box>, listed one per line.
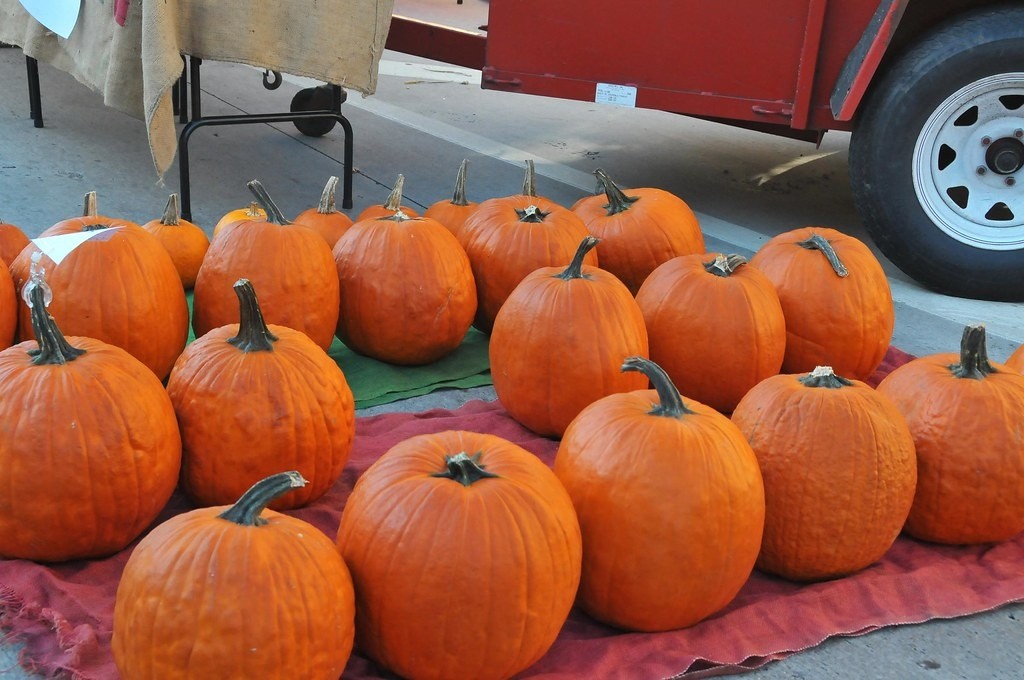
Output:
<box><xmin>0</xmin><ymin>0</ymin><xmax>393</xmax><ymax>224</ymax></box>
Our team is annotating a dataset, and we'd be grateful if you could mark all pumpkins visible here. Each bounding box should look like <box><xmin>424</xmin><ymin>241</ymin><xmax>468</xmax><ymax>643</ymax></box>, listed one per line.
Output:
<box><xmin>0</xmin><ymin>157</ymin><xmax>1024</xmax><ymax>680</ymax></box>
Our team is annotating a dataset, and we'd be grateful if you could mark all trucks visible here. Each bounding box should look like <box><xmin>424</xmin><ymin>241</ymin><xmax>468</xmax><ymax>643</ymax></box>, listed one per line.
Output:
<box><xmin>384</xmin><ymin>0</ymin><xmax>1023</xmax><ymax>302</ymax></box>
